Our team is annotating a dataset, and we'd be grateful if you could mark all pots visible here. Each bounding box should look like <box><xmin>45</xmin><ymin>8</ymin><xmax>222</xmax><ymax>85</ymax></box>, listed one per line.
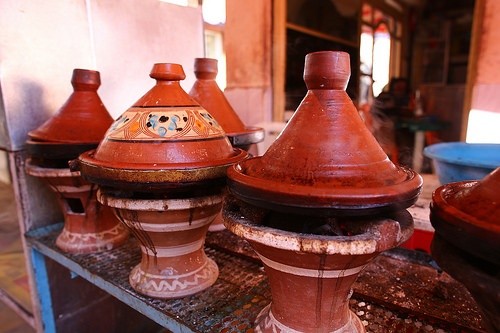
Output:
<box><xmin>191</xmin><ymin>56</ymin><xmax>265</xmax><ymax>146</ymax></box>
<box><xmin>228</xmin><ymin>51</ymin><xmax>424</xmax><ymax>233</ymax></box>
<box><xmin>25</xmin><ymin>68</ymin><xmax>114</xmax><ymax>166</ymax></box>
<box><xmin>79</xmin><ymin>63</ymin><xmax>250</xmax><ymax>198</ymax></box>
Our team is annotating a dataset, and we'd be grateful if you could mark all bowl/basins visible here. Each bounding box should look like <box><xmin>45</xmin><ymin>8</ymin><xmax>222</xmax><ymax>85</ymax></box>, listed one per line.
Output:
<box><xmin>423</xmin><ymin>143</ymin><xmax>499</xmax><ymax>183</ymax></box>
<box><xmin>428</xmin><ymin>180</ymin><xmax>497</xmax><ymax>277</ymax></box>
